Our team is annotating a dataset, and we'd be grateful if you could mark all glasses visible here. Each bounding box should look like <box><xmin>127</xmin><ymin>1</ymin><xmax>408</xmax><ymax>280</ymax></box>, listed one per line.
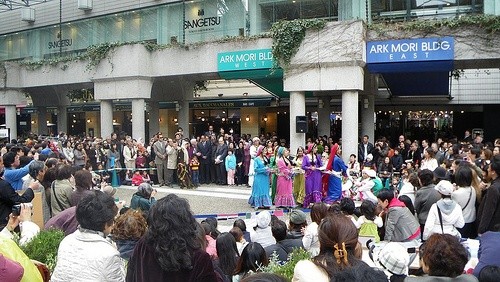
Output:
<box><xmin>160</xmin><ymin>137</ymin><xmax>164</xmax><ymax>139</ymax></box>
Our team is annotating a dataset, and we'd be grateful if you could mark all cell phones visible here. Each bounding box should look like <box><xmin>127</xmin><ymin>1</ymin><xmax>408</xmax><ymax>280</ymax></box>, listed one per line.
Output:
<box><xmin>463</xmin><ymin>157</ymin><xmax>468</xmax><ymax>161</ymax></box>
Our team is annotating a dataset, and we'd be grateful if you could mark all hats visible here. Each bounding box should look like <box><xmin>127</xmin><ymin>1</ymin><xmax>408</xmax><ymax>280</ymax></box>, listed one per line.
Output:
<box><xmin>138</xmin><ymin>182</ymin><xmax>153</xmax><ymax>198</ymax></box>
<box><xmin>103</xmin><ymin>185</ymin><xmax>118</xmax><ymax>197</ymax></box>
<box><xmin>379</xmin><ymin>242</ymin><xmax>411</xmax><ymax>274</ymax></box>
<box><xmin>365</xmin><ymin>169</ymin><xmax>377</xmax><ymax>179</ymax></box>
<box><xmin>256</xmin><ymin>210</ymin><xmax>272</xmax><ymax>229</ymax></box>
<box><xmin>434</xmin><ymin>179</ymin><xmax>454</xmax><ymax>196</ymax></box>
<box><xmin>289</xmin><ymin>209</ymin><xmax>307</xmax><ymax>224</ymax></box>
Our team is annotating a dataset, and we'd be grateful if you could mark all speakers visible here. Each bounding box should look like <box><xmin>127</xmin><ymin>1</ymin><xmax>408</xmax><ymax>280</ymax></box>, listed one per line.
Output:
<box><xmin>295</xmin><ymin>116</ymin><xmax>308</xmax><ymax>133</ymax></box>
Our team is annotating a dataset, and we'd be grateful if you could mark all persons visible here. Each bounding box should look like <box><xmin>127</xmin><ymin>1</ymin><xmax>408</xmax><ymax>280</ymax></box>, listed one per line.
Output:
<box><xmin>0</xmin><ymin>124</ymin><xmax>500</xmax><ymax>282</ymax></box>
<box><xmin>48</xmin><ymin>190</ymin><xmax>126</xmax><ymax>282</ymax></box>
<box><xmin>301</xmin><ymin>143</ymin><xmax>324</xmax><ymax>209</ymax></box>
<box><xmin>273</xmin><ymin>147</ymin><xmax>297</xmax><ymax>209</ymax></box>
<box><xmin>291</xmin><ymin>211</ymin><xmax>374</xmax><ymax>282</ymax></box>
<box><xmin>225</xmin><ymin>148</ymin><xmax>237</xmax><ymax>187</ymax></box>
<box><xmin>124</xmin><ymin>192</ymin><xmax>217</xmax><ymax>282</ymax></box>
<box><xmin>247</xmin><ymin>146</ymin><xmax>275</xmax><ymax>209</ymax></box>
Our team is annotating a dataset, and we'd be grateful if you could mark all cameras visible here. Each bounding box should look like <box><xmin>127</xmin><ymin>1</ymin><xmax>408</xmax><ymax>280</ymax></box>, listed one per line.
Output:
<box><xmin>12</xmin><ymin>203</ymin><xmax>21</xmax><ymax>216</ymax></box>
